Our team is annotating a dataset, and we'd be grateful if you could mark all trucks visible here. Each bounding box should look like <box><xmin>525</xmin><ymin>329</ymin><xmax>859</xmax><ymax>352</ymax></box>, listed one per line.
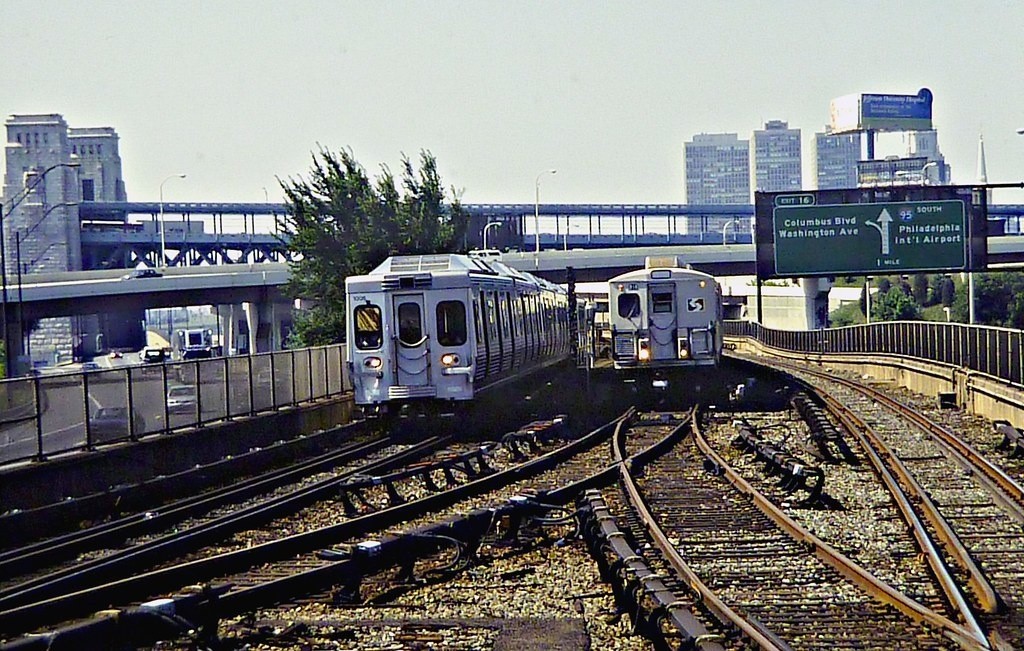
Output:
<box><xmin>140</xmin><ymin>345</ymin><xmax>170</xmax><ymax>372</ymax></box>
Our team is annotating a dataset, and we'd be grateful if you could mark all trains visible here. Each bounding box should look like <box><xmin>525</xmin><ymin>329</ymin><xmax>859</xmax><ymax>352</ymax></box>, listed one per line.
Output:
<box><xmin>343</xmin><ymin>253</ymin><xmax>594</xmax><ymax>420</ymax></box>
<box><xmin>606</xmin><ymin>254</ymin><xmax>725</xmax><ymax>370</ymax></box>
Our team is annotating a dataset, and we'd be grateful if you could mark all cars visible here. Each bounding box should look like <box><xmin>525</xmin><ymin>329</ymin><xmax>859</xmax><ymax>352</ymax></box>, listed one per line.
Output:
<box><xmin>88</xmin><ymin>406</ymin><xmax>144</xmax><ymax>442</ymax></box>
<box><xmin>167</xmin><ymin>385</ymin><xmax>199</xmax><ymax>412</ymax></box>
<box><xmin>80</xmin><ymin>361</ymin><xmax>104</xmax><ymax>376</ymax></box>
<box><xmin>121</xmin><ymin>268</ymin><xmax>165</xmax><ymax>280</ymax></box>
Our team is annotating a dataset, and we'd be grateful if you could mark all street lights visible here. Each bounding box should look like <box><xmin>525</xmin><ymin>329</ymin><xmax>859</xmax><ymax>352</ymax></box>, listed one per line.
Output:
<box><xmin>158</xmin><ymin>173</ymin><xmax>188</xmax><ymax>269</ymax></box>
<box><xmin>921</xmin><ymin>162</ymin><xmax>937</xmax><ymax>187</ymax></box>
<box><xmin>564</xmin><ymin>224</ymin><xmax>581</xmax><ymax>251</ymax></box>
<box><xmin>535</xmin><ymin>168</ymin><xmax>558</xmax><ymax>251</ymax></box>
<box><xmin>0</xmin><ymin>163</ymin><xmax>85</xmax><ymax>407</ymax></box>
<box><xmin>15</xmin><ymin>202</ymin><xmax>78</xmax><ymax>357</ymax></box>
<box><xmin>23</xmin><ymin>239</ymin><xmax>67</xmax><ymax>355</ymax></box>
<box><xmin>722</xmin><ymin>221</ymin><xmax>744</xmax><ymax>246</ymax></box>
<box><xmin>485</xmin><ymin>221</ymin><xmax>502</xmax><ymax>258</ymax></box>
